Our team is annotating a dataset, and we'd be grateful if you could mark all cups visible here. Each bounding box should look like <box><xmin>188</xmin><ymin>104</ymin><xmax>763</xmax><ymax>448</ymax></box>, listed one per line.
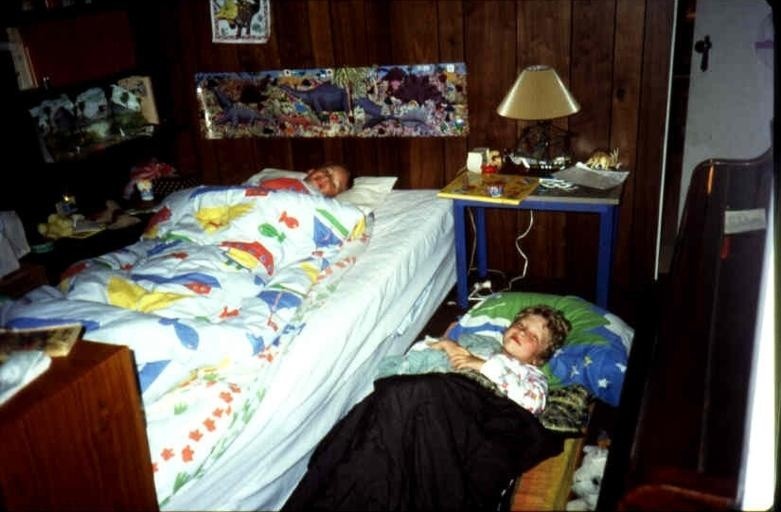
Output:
<box><xmin>137</xmin><ymin>179</ymin><xmax>154</xmax><ymax>201</ymax></box>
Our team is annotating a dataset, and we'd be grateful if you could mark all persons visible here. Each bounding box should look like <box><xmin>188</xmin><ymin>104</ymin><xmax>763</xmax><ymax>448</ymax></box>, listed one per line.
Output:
<box><xmin>409</xmin><ymin>303</ymin><xmax>571</xmax><ymax>418</ymax></box>
<box><xmin>306</xmin><ymin>161</ymin><xmax>354</xmax><ymax>199</ymax></box>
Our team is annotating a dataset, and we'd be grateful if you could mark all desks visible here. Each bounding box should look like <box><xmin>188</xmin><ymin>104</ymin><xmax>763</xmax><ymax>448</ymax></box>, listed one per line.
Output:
<box><xmin>437</xmin><ymin>168</ymin><xmax>628</xmax><ymax>314</ymax></box>
<box><xmin>1</xmin><ymin>338</ymin><xmax>161</xmax><ymax>510</ymax></box>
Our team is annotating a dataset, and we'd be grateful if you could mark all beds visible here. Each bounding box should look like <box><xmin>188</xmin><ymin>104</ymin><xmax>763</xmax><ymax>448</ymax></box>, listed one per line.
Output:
<box><xmin>43</xmin><ymin>168</ymin><xmax>466</xmax><ymax>512</ymax></box>
<box><xmin>287</xmin><ymin>293</ymin><xmax>644</xmax><ymax>511</ymax></box>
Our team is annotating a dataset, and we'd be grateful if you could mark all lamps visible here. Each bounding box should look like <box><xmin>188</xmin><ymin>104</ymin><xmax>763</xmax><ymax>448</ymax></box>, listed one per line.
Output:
<box><xmin>494</xmin><ymin>62</ymin><xmax>583</xmax><ymax>175</ymax></box>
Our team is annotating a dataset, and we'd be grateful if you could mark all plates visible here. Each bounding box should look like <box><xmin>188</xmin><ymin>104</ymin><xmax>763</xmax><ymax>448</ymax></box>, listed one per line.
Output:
<box><xmin>550</xmin><ymin>162</ymin><xmax>632</xmax><ymax>191</ymax></box>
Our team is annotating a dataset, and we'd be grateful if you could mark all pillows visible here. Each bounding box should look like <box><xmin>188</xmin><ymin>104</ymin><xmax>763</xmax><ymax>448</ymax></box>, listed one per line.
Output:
<box><xmin>240</xmin><ymin>164</ymin><xmax>400</xmax><ymax>215</ymax></box>
<box><xmin>448</xmin><ymin>292</ymin><xmax>637</xmax><ymax>406</ymax></box>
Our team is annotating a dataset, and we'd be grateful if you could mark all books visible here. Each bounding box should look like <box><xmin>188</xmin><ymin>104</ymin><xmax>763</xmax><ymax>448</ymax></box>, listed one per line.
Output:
<box><xmin>0</xmin><ymin>321</ymin><xmax>84</xmax><ymax>358</ymax></box>
